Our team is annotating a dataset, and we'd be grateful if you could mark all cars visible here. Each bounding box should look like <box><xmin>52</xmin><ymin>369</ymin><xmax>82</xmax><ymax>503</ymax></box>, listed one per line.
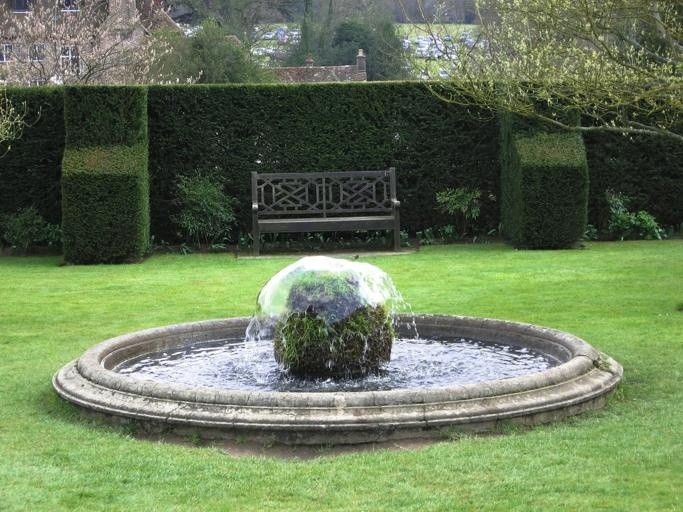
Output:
<box><xmin>395</xmin><ymin>28</ymin><xmax>493</xmax><ymax>82</ymax></box>
<box><xmin>245</xmin><ymin>22</ymin><xmax>303</xmax><ymax>69</ymax></box>
